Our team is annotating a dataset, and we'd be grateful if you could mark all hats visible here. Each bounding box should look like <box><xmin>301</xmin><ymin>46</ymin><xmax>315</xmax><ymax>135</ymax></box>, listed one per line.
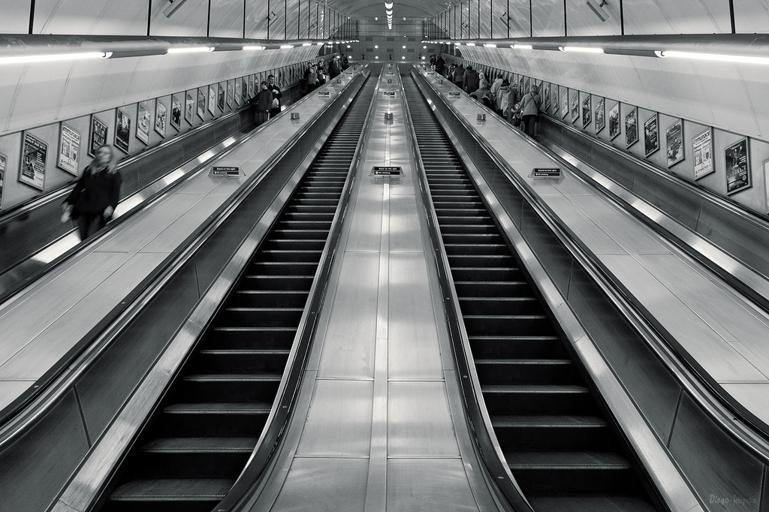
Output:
<box><xmin>501</xmin><ymin>79</ymin><xmax>510</xmax><ymax>87</ymax></box>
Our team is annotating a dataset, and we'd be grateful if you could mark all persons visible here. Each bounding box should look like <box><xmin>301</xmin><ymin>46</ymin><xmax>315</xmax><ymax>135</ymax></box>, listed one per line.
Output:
<box><xmin>246</xmin><ymin>74</ymin><xmax>283</xmax><ymax>127</ymax></box>
<box><xmin>304</xmin><ymin>53</ymin><xmax>350</xmax><ymax>97</ymax></box>
<box><xmin>59</xmin><ymin>144</ymin><xmax>123</xmax><ymax>243</ymax></box>
<box><xmin>429</xmin><ymin>54</ymin><xmax>541</xmax><ymax>140</ymax></box>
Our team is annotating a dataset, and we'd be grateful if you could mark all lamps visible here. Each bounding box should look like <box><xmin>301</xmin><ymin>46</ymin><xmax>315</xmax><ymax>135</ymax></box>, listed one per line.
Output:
<box><xmin>384</xmin><ymin>0</ymin><xmax>394</xmax><ymax>15</ymax></box>
<box><xmin>0</xmin><ymin>40</ymin><xmax>324</xmax><ymax>65</ymax></box>
<box><xmin>587</xmin><ymin>0</ymin><xmax>609</xmax><ymax>22</ymax></box>
<box><xmin>453</xmin><ymin>41</ymin><xmax>769</xmax><ymax>66</ymax></box>
<box><xmin>162</xmin><ymin>0</ymin><xmax>185</xmax><ymax>17</ymax></box>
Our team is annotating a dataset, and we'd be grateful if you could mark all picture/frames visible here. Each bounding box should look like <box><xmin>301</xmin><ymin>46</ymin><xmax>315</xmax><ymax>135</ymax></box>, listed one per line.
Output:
<box><xmin>518</xmin><ymin>74</ymin><xmax>769</xmax><ymax>215</ymax></box>
<box><xmin>0</xmin><ymin>68</ymin><xmax>280</xmax><ymax>211</ymax></box>
